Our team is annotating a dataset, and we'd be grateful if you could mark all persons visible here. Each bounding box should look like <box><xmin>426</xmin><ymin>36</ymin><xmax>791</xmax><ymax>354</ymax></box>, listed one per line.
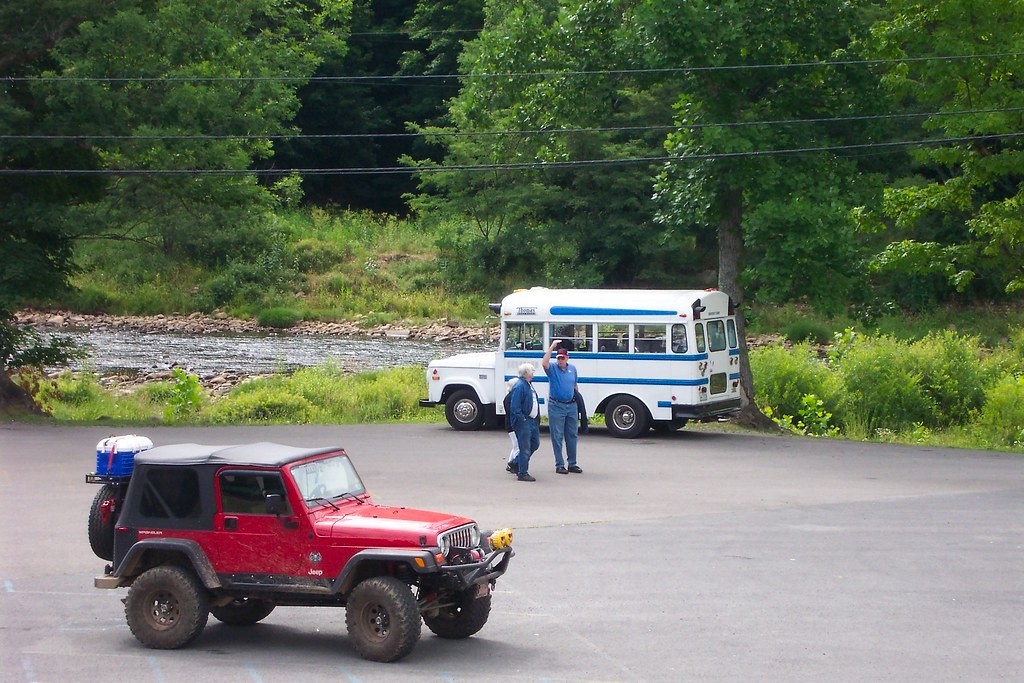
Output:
<box><xmin>542</xmin><ymin>340</ymin><xmax>583</xmax><ymax>474</ymax></box>
<box><xmin>503</xmin><ymin>363</ymin><xmax>540</xmax><ymax>481</ymax></box>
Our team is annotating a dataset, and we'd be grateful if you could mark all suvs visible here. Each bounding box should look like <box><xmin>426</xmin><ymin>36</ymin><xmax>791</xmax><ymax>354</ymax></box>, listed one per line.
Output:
<box><xmin>86</xmin><ymin>435</ymin><xmax>516</xmax><ymax>662</ymax></box>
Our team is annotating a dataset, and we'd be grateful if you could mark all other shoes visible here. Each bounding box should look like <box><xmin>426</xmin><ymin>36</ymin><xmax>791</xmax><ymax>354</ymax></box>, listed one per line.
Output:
<box><xmin>518</xmin><ymin>473</ymin><xmax>536</xmax><ymax>481</ymax></box>
<box><xmin>556</xmin><ymin>467</ymin><xmax>568</xmax><ymax>473</ymax></box>
<box><xmin>568</xmin><ymin>465</ymin><xmax>582</xmax><ymax>472</ymax></box>
<box><xmin>506</xmin><ymin>462</ymin><xmax>518</xmax><ymax>473</ymax></box>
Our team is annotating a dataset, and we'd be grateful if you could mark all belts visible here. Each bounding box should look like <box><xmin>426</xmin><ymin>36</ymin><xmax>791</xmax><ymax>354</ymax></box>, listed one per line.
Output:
<box><xmin>550</xmin><ymin>397</ymin><xmax>575</xmax><ymax>403</ymax></box>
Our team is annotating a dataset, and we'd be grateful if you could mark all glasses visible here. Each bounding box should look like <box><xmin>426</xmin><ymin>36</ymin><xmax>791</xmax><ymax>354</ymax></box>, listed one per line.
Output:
<box><xmin>557</xmin><ymin>356</ymin><xmax>565</xmax><ymax>360</ymax></box>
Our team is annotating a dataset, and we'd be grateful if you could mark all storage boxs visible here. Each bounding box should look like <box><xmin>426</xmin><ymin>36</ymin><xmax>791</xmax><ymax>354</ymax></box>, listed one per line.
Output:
<box><xmin>96</xmin><ymin>434</ymin><xmax>153</xmax><ymax>481</ymax></box>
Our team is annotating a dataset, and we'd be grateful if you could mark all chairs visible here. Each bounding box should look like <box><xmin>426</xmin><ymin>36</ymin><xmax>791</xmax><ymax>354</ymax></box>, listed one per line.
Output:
<box><xmin>587</xmin><ymin>334</ymin><xmax>663</xmax><ymax>353</ymax></box>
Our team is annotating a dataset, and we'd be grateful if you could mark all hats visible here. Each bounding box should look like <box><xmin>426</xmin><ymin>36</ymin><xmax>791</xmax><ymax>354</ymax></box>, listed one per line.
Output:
<box><xmin>556</xmin><ymin>349</ymin><xmax>567</xmax><ymax>357</ymax></box>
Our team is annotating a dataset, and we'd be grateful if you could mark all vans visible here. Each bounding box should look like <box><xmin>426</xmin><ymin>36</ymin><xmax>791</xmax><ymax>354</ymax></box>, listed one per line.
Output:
<box><xmin>418</xmin><ymin>286</ymin><xmax>743</xmax><ymax>440</ymax></box>
